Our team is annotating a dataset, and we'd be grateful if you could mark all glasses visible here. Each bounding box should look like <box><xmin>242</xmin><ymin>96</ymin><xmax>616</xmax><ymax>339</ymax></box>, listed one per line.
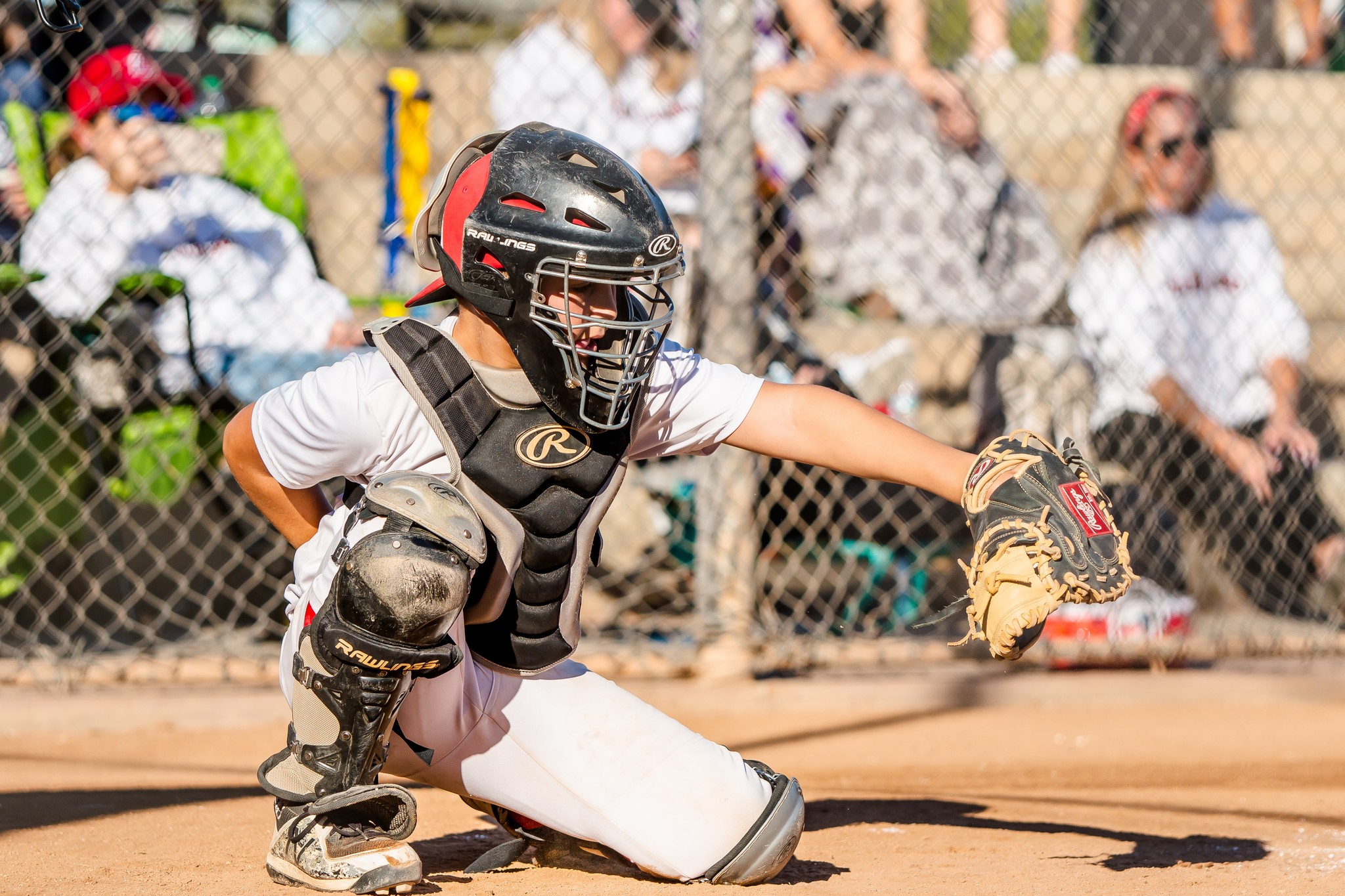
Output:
<box><xmin>1145</xmin><ymin>122</ymin><xmax>1213</xmax><ymax>157</ymax></box>
<box><xmin>628</xmin><ymin>0</ymin><xmax>678</xmax><ymax>48</ymax></box>
<box><xmin>107</xmin><ymin>104</ymin><xmax>180</xmax><ymax>126</ymax></box>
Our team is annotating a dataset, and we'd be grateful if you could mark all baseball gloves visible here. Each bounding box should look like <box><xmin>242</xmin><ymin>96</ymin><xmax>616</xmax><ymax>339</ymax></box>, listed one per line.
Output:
<box><xmin>958</xmin><ymin>437</ymin><xmax>1130</xmax><ymax>660</ymax></box>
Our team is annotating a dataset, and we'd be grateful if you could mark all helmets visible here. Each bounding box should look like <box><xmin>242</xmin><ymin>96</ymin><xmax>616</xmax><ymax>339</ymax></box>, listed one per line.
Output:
<box><xmin>413</xmin><ymin>119</ymin><xmax>685</xmax><ymax>435</ymax></box>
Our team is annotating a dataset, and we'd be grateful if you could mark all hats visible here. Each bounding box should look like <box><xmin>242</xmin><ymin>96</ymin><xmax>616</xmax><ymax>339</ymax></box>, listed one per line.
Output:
<box><xmin>67</xmin><ymin>44</ymin><xmax>179</xmax><ymax>123</ymax></box>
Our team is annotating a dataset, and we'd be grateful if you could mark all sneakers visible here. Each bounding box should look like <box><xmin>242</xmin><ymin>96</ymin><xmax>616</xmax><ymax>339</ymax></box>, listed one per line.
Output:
<box><xmin>266</xmin><ymin>796</ymin><xmax>422</xmax><ymax>895</ymax></box>
<box><xmin>460</xmin><ymin>795</ymin><xmax>551</xmax><ymax>842</ymax></box>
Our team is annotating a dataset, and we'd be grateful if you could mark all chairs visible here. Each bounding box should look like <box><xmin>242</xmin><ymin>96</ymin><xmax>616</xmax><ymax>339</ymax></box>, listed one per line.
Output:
<box><xmin>3</xmin><ymin>74</ymin><xmax>1085</xmax><ymax>653</ymax></box>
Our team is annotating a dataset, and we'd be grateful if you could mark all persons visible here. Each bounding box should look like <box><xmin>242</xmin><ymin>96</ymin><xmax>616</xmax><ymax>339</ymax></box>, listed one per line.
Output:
<box><xmin>489</xmin><ymin>0</ymin><xmax>701</xmax><ymax>190</ymax></box>
<box><xmin>1214</xmin><ymin>0</ymin><xmax>1330</xmax><ymax>71</ymax></box>
<box><xmin>1068</xmin><ymin>85</ymin><xmax>1345</xmax><ymax>625</ymax></box>
<box><xmin>960</xmin><ymin>0</ymin><xmax>1088</xmax><ymax>76</ymax></box>
<box><xmin>748</xmin><ymin>0</ymin><xmax>976</xmax><ymax>176</ymax></box>
<box><xmin>222</xmin><ymin>121</ymin><xmax>1130</xmax><ymax>895</ymax></box>
<box><xmin>21</xmin><ymin>44</ymin><xmax>369</xmax><ymax>402</ymax></box>
